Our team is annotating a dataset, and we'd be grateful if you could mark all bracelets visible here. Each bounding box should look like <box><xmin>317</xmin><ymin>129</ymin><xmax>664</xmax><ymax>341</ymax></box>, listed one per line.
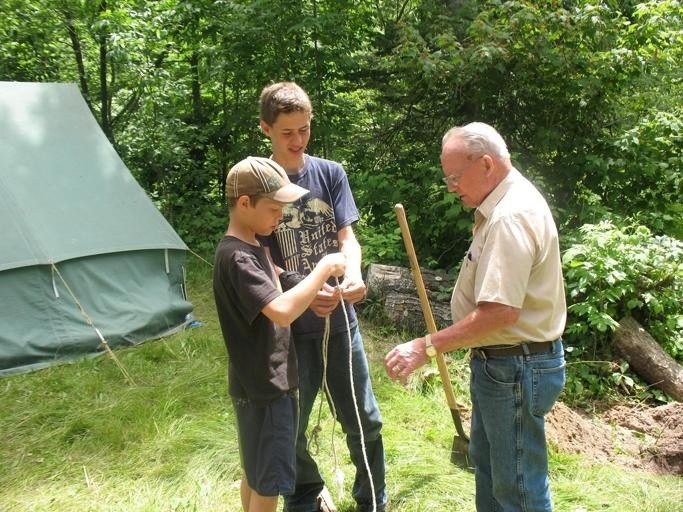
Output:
<box><xmin>280</xmin><ymin>270</ymin><xmax>307</xmax><ymax>291</ymax></box>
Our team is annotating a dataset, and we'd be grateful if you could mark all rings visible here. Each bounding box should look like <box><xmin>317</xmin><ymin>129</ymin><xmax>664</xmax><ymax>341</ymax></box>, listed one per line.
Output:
<box><xmin>396</xmin><ymin>365</ymin><xmax>401</xmax><ymax>372</ymax></box>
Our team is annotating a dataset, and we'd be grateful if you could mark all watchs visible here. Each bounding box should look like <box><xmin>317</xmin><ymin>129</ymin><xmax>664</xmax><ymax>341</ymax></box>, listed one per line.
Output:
<box><xmin>425</xmin><ymin>333</ymin><xmax>438</xmax><ymax>364</ymax></box>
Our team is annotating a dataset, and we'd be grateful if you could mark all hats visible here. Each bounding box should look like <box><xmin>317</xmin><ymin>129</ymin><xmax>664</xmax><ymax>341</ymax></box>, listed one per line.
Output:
<box><xmin>223</xmin><ymin>155</ymin><xmax>311</xmax><ymax>204</ymax></box>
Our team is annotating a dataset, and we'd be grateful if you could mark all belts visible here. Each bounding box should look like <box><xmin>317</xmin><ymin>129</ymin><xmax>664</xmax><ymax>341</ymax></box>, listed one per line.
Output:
<box><xmin>470</xmin><ymin>338</ymin><xmax>553</xmax><ymax>357</ymax></box>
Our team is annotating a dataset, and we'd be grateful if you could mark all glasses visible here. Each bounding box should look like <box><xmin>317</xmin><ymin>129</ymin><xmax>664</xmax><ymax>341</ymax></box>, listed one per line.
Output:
<box><xmin>442</xmin><ymin>174</ymin><xmax>461</xmax><ymax>186</ymax></box>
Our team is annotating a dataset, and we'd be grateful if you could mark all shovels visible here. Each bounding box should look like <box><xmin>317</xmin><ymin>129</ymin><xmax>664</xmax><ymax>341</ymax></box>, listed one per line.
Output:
<box><xmin>394</xmin><ymin>201</ymin><xmax>479</xmax><ymax>474</ymax></box>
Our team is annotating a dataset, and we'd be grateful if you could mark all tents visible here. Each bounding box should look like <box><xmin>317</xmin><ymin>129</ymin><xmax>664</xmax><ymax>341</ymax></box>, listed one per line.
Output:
<box><xmin>0</xmin><ymin>81</ymin><xmax>196</xmax><ymax>378</ymax></box>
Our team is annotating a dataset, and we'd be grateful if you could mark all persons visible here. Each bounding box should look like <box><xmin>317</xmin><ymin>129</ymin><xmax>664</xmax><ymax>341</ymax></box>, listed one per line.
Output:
<box><xmin>384</xmin><ymin>121</ymin><xmax>567</xmax><ymax>512</ymax></box>
<box><xmin>260</xmin><ymin>81</ymin><xmax>387</xmax><ymax>512</ymax></box>
<box><xmin>213</xmin><ymin>155</ymin><xmax>348</xmax><ymax>512</ymax></box>
